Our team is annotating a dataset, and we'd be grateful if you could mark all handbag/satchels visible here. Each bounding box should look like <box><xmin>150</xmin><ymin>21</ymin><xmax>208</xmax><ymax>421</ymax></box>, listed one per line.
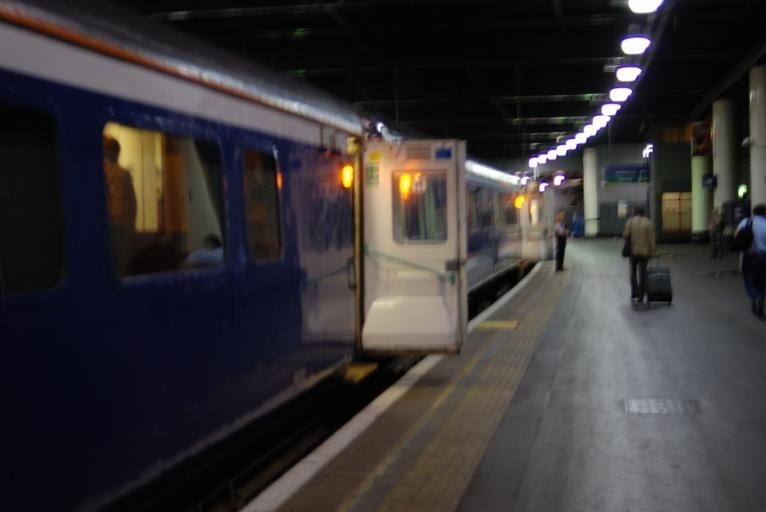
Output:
<box><xmin>622</xmin><ymin>233</ymin><xmax>631</xmax><ymax>257</ymax></box>
<box><xmin>735</xmin><ymin>217</ymin><xmax>754</xmax><ymax>252</ymax></box>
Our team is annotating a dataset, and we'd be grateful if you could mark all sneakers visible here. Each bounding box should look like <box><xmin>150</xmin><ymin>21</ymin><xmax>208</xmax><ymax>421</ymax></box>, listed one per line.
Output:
<box><xmin>751</xmin><ymin>304</ymin><xmax>766</xmax><ymax>321</ymax></box>
<box><xmin>555</xmin><ymin>268</ymin><xmax>567</xmax><ymax>272</ymax></box>
<box><xmin>630</xmin><ymin>292</ymin><xmax>643</xmax><ymax>305</ymax></box>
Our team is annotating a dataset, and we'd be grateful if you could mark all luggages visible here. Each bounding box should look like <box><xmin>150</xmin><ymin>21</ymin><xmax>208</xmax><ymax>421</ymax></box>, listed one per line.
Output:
<box><xmin>645</xmin><ymin>256</ymin><xmax>672</xmax><ymax>307</ymax></box>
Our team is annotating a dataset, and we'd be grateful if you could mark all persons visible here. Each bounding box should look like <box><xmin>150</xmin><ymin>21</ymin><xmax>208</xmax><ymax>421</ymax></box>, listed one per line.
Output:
<box><xmin>102</xmin><ymin>135</ymin><xmax>138</xmax><ymax>277</ymax></box>
<box><xmin>705</xmin><ymin>198</ymin><xmax>754</xmax><ymax>260</ymax></box>
<box><xmin>183</xmin><ymin>234</ymin><xmax>224</xmax><ymax>268</ymax></box>
<box><xmin>623</xmin><ymin>208</ymin><xmax>657</xmax><ymax>304</ymax></box>
<box><xmin>734</xmin><ymin>203</ymin><xmax>765</xmax><ymax>320</ymax></box>
<box><xmin>555</xmin><ymin>210</ymin><xmax>567</xmax><ymax>272</ymax></box>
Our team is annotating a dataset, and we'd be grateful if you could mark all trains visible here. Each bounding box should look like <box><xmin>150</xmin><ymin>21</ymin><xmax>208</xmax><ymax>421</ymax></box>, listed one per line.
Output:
<box><xmin>2</xmin><ymin>0</ymin><xmax>540</xmax><ymax>507</ymax></box>
